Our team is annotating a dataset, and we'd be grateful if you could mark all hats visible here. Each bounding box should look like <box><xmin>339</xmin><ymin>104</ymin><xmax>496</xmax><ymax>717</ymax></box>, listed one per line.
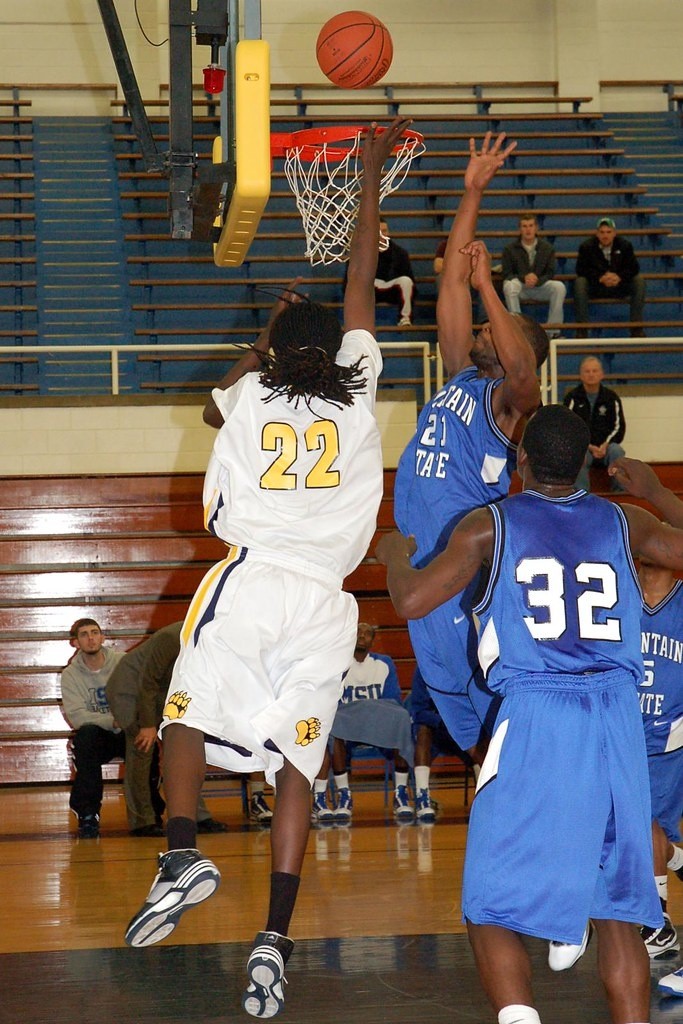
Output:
<box><xmin>597</xmin><ymin>218</ymin><xmax>616</xmax><ymax>229</ymax></box>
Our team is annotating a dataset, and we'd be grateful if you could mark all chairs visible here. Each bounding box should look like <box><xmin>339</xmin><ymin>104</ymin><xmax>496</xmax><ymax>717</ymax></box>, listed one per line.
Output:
<box><xmin>203</xmin><ymin>692</ymin><xmax>483</xmax><ymax>818</ymax></box>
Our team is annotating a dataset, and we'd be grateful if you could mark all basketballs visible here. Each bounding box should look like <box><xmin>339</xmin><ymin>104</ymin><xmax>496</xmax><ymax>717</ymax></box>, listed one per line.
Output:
<box><xmin>316</xmin><ymin>10</ymin><xmax>393</xmax><ymax>90</ymax></box>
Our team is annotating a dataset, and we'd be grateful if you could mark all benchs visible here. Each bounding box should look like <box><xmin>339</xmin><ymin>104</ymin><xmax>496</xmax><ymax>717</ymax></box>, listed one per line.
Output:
<box><xmin>0</xmin><ymin>78</ymin><xmax>683</xmax><ymax>395</ymax></box>
<box><xmin>0</xmin><ymin>460</ymin><xmax>683</xmax><ymax>784</ymax></box>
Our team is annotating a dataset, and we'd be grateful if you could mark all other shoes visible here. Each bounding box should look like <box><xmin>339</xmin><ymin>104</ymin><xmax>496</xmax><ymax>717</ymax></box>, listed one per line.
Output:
<box><xmin>199</xmin><ymin>816</ymin><xmax>226</xmax><ymax>830</ymax></box>
<box><xmin>133</xmin><ymin>825</ymin><xmax>167</xmax><ymax>837</ymax></box>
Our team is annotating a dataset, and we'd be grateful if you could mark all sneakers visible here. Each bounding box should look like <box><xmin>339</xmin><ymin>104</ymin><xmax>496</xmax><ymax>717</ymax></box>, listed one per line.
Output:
<box><xmin>546</xmin><ymin>923</ymin><xmax>593</xmax><ymax>972</ymax></box>
<box><xmin>78</xmin><ymin>814</ymin><xmax>101</xmax><ymax>836</ymax></box>
<box><xmin>248</xmin><ymin>825</ymin><xmax>272</xmax><ymax>843</ymax></box>
<box><xmin>413</xmin><ymin>787</ymin><xmax>437</xmax><ymax>820</ymax></box>
<box><xmin>657</xmin><ymin>967</ymin><xmax>683</xmax><ymax>996</ymax></box>
<box><xmin>124</xmin><ymin>849</ymin><xmax>223</xmax><ymax>947</ymax></box>
<box><xmin>390</xmin><ymin>784</ymin><xmax>414</xmax><ymax>817</ymax></box>
<box><xmin>334</xmin><ymin>787</ymin><xmax>354</xmax><ymax>818</ymax></box>
<box><xmin>636</xmin><ymin>907</ymin><xmax>680</xmax><ymax>959</ymax></box>
<box><xmin>249</xmin><ymin>794</ymin><xmax>275</xmax><ymax>822</ymax></box>
<box><xmin>311</xmin><ymin>792</ymin><xmax>334</xmax><ymax>820</ymax></box>
<box><xmin>242</xmin><ymin>931</ymin><xmax>296</xmax><ymax>1019</ymax></box>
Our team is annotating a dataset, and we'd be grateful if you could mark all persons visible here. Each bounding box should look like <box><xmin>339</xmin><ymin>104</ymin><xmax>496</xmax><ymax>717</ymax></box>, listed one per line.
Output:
<box><xmin>374</xmin><ymin>406</ymin><xmax>683</xmax><ymax>1024</ymax></box>
<box><xmin>393</xmin><ymin>133</ymin><xmax>591</xmax><ymax>970</ymax></box>
<box><xmin>60</xmin><ymin>618</ymin><xmax>480</xmax><ymax>838</ymax></box>
<box><xmin>123</xmin><ymin>112</ymin><xmax>415</xmax><ymax>1020</ymax></box>
<box><xmin>341</xmin><ymin>218</ymin><xmax>646</xmax><ymax>492</ymax></box>
<box><xmin>636</xmin><ymin>519</ymin><xmax>683</xmax><ymax>996</ymax></box>
